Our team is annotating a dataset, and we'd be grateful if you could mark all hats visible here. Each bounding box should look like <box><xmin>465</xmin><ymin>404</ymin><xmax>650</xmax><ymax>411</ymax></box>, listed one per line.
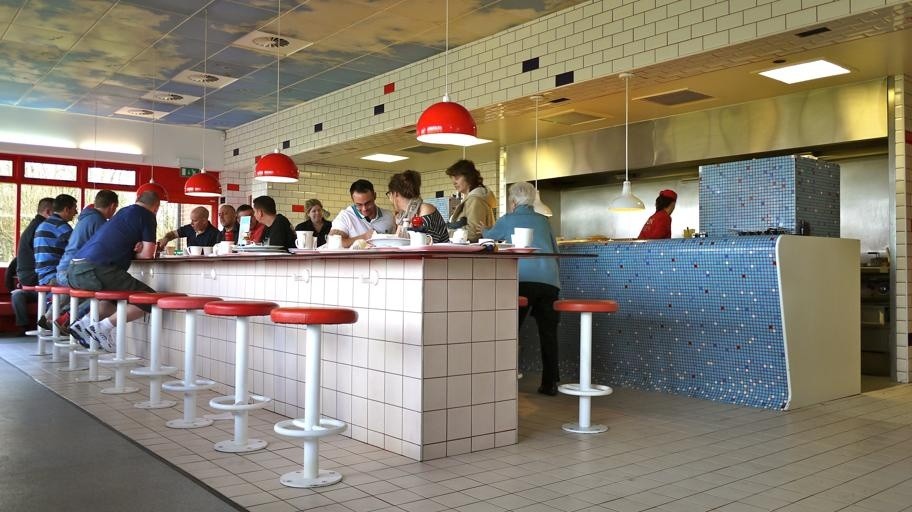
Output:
<box><xmin>660</xmin><ymin>190</ymin><xmax>677</xmax><ymax>199</ymax></box>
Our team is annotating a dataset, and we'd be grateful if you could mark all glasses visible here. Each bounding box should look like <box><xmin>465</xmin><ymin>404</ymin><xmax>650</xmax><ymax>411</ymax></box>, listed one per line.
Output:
<box><xmin>385</xmin><ymin>191</ymin><xmax>391</xmax><ymax>198</ymax></box>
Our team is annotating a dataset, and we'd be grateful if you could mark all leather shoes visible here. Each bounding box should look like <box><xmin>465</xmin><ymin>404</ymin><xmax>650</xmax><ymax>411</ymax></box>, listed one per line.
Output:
<box><xmin>537</xmin><ymin>383</ymin><xmax>559</xmax><ymax>395</ymax></box>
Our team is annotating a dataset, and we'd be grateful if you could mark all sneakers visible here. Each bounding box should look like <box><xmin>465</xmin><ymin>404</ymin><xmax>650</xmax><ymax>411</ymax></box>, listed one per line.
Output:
<box><xmin>66</xmin><ymin>320</ymin><xmax>91</xmax><ymax>347</ymax></box>
<box><xmin>85</xmin><ymin>322</ymin><xmax>116</xmax><ymax>353</ymax></box>
<box><xmin>54</xmin><ymin>312</ymin><xmax>71</xmax><ymax>334</ymax></box>
<box><xmin>38</xmin><ymin>314</ymin><xmax>53</xmax><ymax>330</ymax></box>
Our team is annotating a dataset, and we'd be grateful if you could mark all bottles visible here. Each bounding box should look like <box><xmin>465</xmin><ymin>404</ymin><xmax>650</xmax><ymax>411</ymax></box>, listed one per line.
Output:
<box><xmin>800</xmin><ymin>219</ymin><xmax>806</xmax><ymax>235</ymax></box>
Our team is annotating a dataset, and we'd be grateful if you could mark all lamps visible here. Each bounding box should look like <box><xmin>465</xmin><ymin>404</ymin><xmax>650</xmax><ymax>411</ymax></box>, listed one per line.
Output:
<box><xmin>184</xmin><ymin>60</ymin><xmax>223</xmax><ymax>197</ymax></box>
<box><xmin>530</xmin><ymin>95</ymin><xmax>554</xmax><ymax>217</ymax></box>
<box><xmin>255</xmin><ymin>41</ymin><xmax>300</xmax><ymax>183</ymax></box>
<box><xmin>137</xmin><ymin>107</ymin><xmax>171</xmax><ymax>202</ymax></box>
<box><xmin>612</xmin><ymin>75</ymin><xmax>646</xmax><ymax>209</ymax></box>
<box><xmin>416</xmin><ymin>0</ymin><xmax>477</xmax><ymax>143</ymax></box>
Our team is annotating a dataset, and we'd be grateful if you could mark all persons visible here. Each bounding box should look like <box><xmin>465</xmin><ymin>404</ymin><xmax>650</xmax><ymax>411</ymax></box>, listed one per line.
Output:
<box><xmin>17</xmin><ymin>198</ymin><xmax>55</xmax><ymax>286</ymax></box>
<box><xmin>56</xmin><ymin>191</ymin><xmax>118</xmax><ymax>336</ymax></box>
<box><xmin>33</xmin><ymin>194</ymin><xmax>77</xmax><ymax>330</ymax></box>
<box><xmin>476</xmin><ymin>182</ymin><xmax>562</xmax><ymax>396</ymax></box>
<box><xmin>66</xmin><ymin>190</ymin><xmax>157</xmax><ymax>353</ymax></box>
<box><xmin>217</xmin><ymin>206</ymin><xmax>240</xmax><ymax>245</ymax></box>
<box><xmin>159</xmin><ymin>207</ymin><xmax>220</xmax><ymax>248</ymax></box>
<box><xmin>638</xmin><ymin>190</ymin><xmax>677</xmax><ymax>240</ymax></box>
<box><xmin>445</xmin><ymin>160</ymin><xmax>498</xmax><ymax>243</ymax></box>
<box><xmin>386</xmin><ymin>170</ymin><xmax>449</xmax><ymax>243</ymax></box>
<box><xmin>236</xmin><ymin>204</ymin><xmax>265</xmax><ymax>242</ymax></box>
<box><xmin>253</xmin><ymin>197</ymin><xmax>297</xmax><ymax>249</ymax></box>
<box><xmin>5</xmin><ymin>257</ymin><xmax>38</xmax><ymax>336</ymax></box>
<box><xmin>328</xmin><ymin>179</ymin><xmax>396</xmax><ymax>248</ymax></box>
<box><xmin>295</xmin><ymin>199</ymin><xmax>332</xmax><ymax>247</ymax></box>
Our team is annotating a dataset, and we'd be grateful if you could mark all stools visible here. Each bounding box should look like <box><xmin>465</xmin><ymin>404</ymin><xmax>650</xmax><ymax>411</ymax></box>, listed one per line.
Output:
<box><xmin>95</xmin><ymin>291</ymin><xmax>142</xmax><ymax>394</ymax></box>
<box><xmin>270</xmin><ymin>307</ymin><xmax>357</xmax><ymax>488</ymax></box>
<box><xmin>205</xmin><ymin>302</ymin><xmax>276</xmax><ymax>454</ymax></box>
<box><xmin>23</xmin><ymin>285</ymin><xmax>47</xmax><ymax>359</ymax></box>
<box><xmin>129</xmin><ymin>293</ymin><xmax>186</xmax><ymax>408</ymax></box>
<box><xmin>34</xmin><ymin>286</ymin><xmax>67</xmax><ymax>361</ymax></box>
<box><xmin>52</xmin><ymin>287</ymin><xmax>90</xmax><ymax>372</ymax></box>
<box><xmin>552</xmin><ymin>300</ymin><xmax>618</xmax><ymax>434</ymax></box>
<box><xmin>159</xmin><ymin>298</ymin><xmax>221</xmax><ymax>431</ymax></box>
<box><xmin>68</xmin><ymin>289</ymin><xmax>105</xmax><ymax>384</ymax></box>
<box><xmin>517</xmin><ymin>297</ymin><xmax>529</xmax><ymax>380</ymax></box>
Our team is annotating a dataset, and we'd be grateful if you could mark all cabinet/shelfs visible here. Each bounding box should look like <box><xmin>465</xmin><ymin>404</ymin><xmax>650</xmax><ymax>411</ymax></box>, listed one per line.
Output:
<box><xmin>522</xmin><ymin>237</ymin><xmax>860</xmax><ymax>410</ymax></box>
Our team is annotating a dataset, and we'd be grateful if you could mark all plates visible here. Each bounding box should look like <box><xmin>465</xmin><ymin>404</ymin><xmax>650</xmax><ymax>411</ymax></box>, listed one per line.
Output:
<box><xmin>366</xmin><ymin>238</ymin><xmax>410</xmax><ymax>248</ymax></box>
<box><xmin>469</xmin><ymin>243</ymin><xmax>515</xmax><ymax>249</ymax></box>
<box><xmin>506</xmin><ymin>247</ymin><xmax>542</xmax><ymax>254</ymax></box>
<box><xmin>230</xmin><ymin>245</ymin><xmax>288</xmax><ymax>252</ymax></box>
<box><xmin>289</xmin><ymin>248</ymin><xmax>320</xmax><ymax>254</ymax></box>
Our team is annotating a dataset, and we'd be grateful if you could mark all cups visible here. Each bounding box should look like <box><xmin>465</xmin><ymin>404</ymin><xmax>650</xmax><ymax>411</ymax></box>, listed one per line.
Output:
<box><xmin>325</xmin><ymin>234</ymin><xmax>342</xmax><ymax>250</ymax></box>
<box><xmin>295</xmin><ymin>231</ymin><xmax>318</xmax><ymax>250</ymax></box>
<box><xmin>166</xmin><ymin>246</ymin><xmax>175</xmax><ymax>255</ymax></box>
<box><xmin>479</xmin><ymin>239</ymin><xmax>494</xmax><ymax>244</ymax></box>
<box><xmin>511</xmin><ymin>234</ymin><xmax>515</xmax><ymax>244</ymax></box>
<box><xmin>405</xmin><ymin>231</ymin><xmax>433</xmax><ymax>247</ymax></box>
<box><xmin>187</xmin><ymin>246</ymin><xmax>203</xmax><ymax>255</ymax></box>
<box><xmin>221</xmin><ymin>241</ymin><xmax>234</xmax><ymax>256</ymax></box>
<box><xmin>202</xmin><ymin>247</ymin><xmax>213</xmax><ymax>256</ymax></box>
<box><xmin>514</xmin><ymin>227</ymin><xmax>533</xmax><ymax>249</ymax></box>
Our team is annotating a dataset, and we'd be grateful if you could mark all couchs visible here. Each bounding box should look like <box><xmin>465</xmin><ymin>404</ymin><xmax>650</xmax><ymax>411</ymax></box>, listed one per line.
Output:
<box><xmin>0</xmin><ymin>267</ymin><xmax>40</xmax><ymax>330</ymax></box>
